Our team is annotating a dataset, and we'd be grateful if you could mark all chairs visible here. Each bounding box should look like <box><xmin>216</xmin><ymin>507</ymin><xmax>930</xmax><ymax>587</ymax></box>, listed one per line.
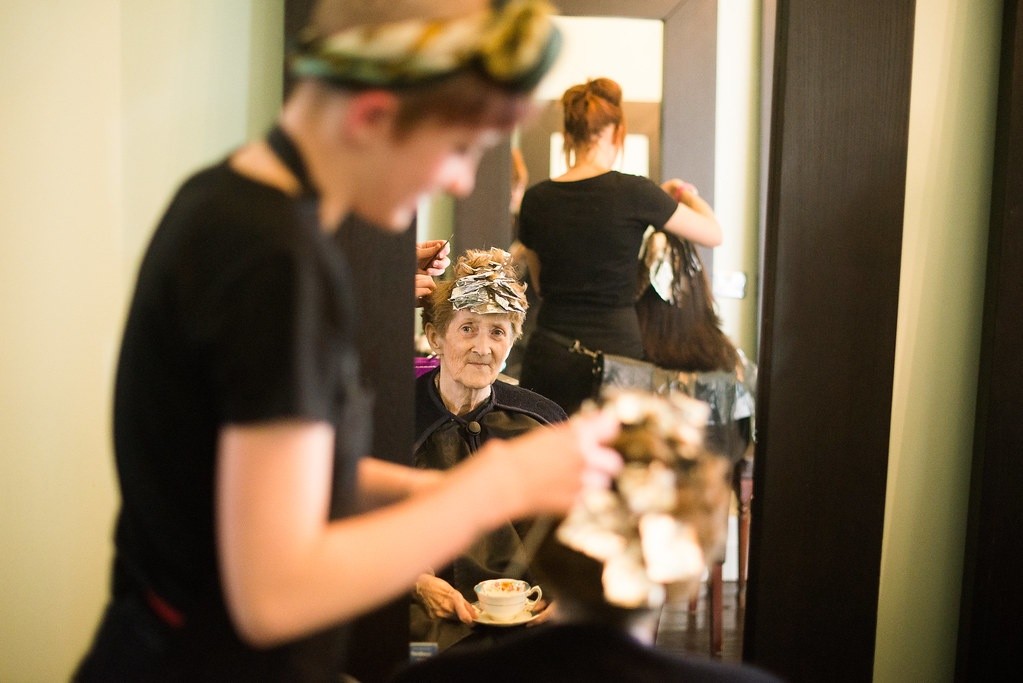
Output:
<box><xmin>681</xmin><ymin>368</ymin><xmax>750</xmax><ymax>660</ymax></box>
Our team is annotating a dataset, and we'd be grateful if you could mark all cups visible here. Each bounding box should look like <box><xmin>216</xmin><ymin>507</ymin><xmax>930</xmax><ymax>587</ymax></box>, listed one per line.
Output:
<box><xmin>474</xmin><ymin>579</ymin><xmax>543</xmax><ymax>621</ymax></box>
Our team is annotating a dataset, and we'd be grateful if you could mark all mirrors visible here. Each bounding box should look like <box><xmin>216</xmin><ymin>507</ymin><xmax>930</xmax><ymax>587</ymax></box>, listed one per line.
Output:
<box><xmin>404</xmin><ymin>0</ymin><xmax>790</xmax><ymax>662</ymax></box>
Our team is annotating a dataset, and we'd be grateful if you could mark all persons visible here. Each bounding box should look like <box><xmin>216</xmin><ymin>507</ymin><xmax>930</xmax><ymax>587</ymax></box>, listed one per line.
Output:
<box><xmin>410</xmin><ymin>78</ymin><xmax>752</xmax><ymax>664</ymax></box>
<box><xmin>67</xmin><ymin>0</ymin><xmax>624</xmax><ymax>683</ymax></box>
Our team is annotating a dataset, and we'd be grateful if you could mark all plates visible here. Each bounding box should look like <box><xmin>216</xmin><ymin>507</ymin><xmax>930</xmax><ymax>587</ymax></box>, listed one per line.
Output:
<box><xmin>470</xmin><ymin>600</ymin><xmax>541</xmax><ymax>627</ymax></box>
